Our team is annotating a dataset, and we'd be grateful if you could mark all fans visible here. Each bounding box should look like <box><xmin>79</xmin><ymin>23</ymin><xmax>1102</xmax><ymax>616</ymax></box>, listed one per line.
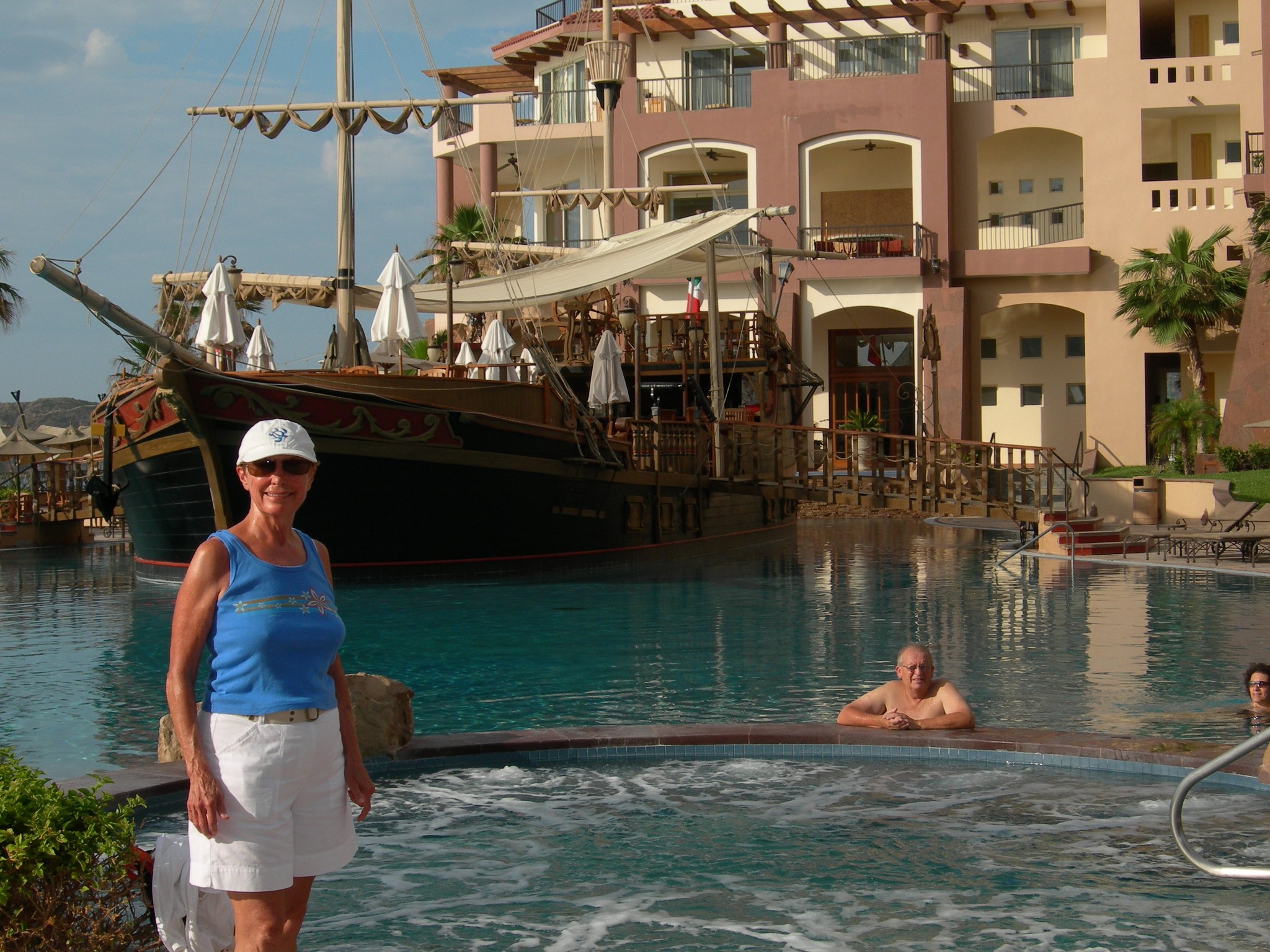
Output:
<box><xmin>691</xmin><ymin>148</ymin><xmax>736</xmax><ymax>162</ymax></box>
<box><xmin>497</xmin><ymin>153</ymin><xmax>522</xmax><ymax>176</ymax></box>
<box><xmin>848</xmin><ymin>140</ymin><xmax>895</xmax><ymax>151</ymax></box>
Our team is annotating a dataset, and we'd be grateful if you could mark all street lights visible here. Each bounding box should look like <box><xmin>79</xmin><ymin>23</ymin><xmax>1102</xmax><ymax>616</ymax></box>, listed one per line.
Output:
<box><xmin>617</xmin><ymin>295</ymin><xmax>641</xmax><ymax>420</ymax></box>
<box><xmin>672</xmin><ymin>325</ymin><xmax>704</xmax><ymax>427</ymax></box>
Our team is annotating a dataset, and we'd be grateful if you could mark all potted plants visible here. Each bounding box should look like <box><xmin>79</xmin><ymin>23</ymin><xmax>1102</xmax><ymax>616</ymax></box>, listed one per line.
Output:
<box><xmin>838</xmin><ymin>407</ymin><xmax>888</xmax><ymax>471</ymax></box>
<box><xmin>427</xmin><ymin>327</ymin><xmax>457</xmax><ymax>362</ymax></box>
<box><xmin>814</xmin><ymin>439</ymin><xmax>823</xmax><ymax>470</ymax></box>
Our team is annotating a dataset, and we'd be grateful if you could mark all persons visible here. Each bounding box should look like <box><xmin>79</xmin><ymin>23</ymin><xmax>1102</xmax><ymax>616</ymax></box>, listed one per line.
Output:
<box><xmin>165</xmin><ymin>419</ymin><xmax>377</xmax><ymax>952</ymax></box>
<box><xmin>1241</xmin><ymin>662</ymin><xmax>1270</xmax><ymax>707</ymax></box>
<box><xmin>838</xmin><ymin>643</ymin><xmax>977</xmax><ymax>732</ymax></box>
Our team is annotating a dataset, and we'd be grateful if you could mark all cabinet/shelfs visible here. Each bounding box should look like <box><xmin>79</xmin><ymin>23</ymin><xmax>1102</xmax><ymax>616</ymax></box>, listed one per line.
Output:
<box><xmin>592</xmin><ymin>94</ymin><xmax>675</xmax><ymax>122</ymax></box>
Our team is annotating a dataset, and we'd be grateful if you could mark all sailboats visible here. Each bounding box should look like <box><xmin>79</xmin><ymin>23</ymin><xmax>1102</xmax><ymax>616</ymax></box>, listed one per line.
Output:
<box><xmin>28</xmin><ymin>0</ymin><xmax>826</xmax><ymax>591</ymax></box>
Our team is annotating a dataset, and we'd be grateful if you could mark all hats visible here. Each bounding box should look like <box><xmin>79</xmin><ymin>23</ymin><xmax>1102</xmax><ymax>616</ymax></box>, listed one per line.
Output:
<box><xmin>236</xmin><ymin>419</ymin><xmax>316</xmax><ymax>467</ymax></box>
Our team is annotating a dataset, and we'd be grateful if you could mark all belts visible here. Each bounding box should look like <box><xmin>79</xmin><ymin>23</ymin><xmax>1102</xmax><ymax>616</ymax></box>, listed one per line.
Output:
<box><xmin>238</xmin><ymin>708</ymin><xmax>326</xmax><ymax>725</ymax></box>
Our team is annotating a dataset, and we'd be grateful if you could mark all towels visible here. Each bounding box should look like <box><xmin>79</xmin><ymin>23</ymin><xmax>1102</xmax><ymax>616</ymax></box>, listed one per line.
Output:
<box><xmin>152</xmin><ymin>832</ymin><xmax>236</xmax><ymax>952</ymax></box>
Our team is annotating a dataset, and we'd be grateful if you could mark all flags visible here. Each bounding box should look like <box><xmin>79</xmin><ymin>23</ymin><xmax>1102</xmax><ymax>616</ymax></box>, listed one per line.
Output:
<box><xmin>685</xmin><ymin>277</ymin><xmax>705</xmax><ymax>328</ymax></box>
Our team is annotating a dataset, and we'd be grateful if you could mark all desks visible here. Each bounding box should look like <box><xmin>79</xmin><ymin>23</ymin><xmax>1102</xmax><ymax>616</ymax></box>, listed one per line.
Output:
<box><xmin>827</xmin><ymin>234</ymin><xmax>905</xmax><ymax>258</ymax></box>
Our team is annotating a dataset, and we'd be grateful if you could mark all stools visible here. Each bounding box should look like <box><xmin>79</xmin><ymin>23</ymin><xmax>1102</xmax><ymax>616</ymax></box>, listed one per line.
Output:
<box><xmin>705</xmin><ymin>103</ymin><xmax>732</xmax><ymax>109</ymax></box>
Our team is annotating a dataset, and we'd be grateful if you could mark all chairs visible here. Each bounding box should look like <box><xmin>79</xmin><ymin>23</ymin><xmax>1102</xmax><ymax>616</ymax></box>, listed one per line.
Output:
<box><xmin>1192</xmin><ymin>532</ymin><xmax>1270</xmax><ymax>565</ymax></box>
<box><xmin>852</xmin><ymin>241</ymin><xmax>878</xmax><ymax>258</ymax></box>
<box><xmin>345</xmin><ymin>364</ymin><xmax>379</xmax><ymax>374</ymax></box>
<box><xmin>880</xmin><ymin>239</ymin><xmax>904</xmax><ymax>257</ymax></box>
<box><xmin>814</xmin><ymin>241</ymin><xmax>843</xmax><ymax>253</ymax></box>
<box><xmin>418</xmin><ymin>368</ymin><xmax>446</xmax><ymax>377</ymax></box>
<box><xmin>1123</xmin><ymin>501</ymin><xmax>1261</xmax><ymax>563</ymax></box>
<box><xmin>903</xmin><ymin>237</ymin><xmax>913</xmax><ymax>257</ymax></box>
<box><xmin>1163</xmin><ymin>503</ymin><xmax>1270</xmax><ymax>563</ymax></box>
<box><xmin>446</xmin><ymin>363</ymin><xmax>469</xmax><ymax>378</ymax></box>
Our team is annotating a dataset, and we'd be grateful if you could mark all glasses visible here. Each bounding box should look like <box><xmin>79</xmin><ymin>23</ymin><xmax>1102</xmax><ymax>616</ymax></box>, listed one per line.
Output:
<box><xmin>1247</xmin><ymin>681</ymin><xmax>1270</xmax><ymax>688</ymax></box>
<box><xmin>899</xmin><ymin>664</ymin><xmax>933</xmax><ymax>674</ymax></box>
<box><xmin>241</xmin><ymin>458</ymin><xmax>314</xmax><ymax>478</ymax></box>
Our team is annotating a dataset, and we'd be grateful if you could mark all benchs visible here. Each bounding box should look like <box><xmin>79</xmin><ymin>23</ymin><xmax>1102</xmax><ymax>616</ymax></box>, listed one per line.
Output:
<box><xmin>467</xmin><ymin>361</ymin><xmax>537</xmax><ymax>382</ymax></box>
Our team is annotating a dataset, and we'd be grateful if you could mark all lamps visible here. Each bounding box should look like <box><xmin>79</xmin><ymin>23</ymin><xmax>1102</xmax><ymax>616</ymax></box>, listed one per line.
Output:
<box><xmin>793</xmin><ymin>53</ymin><xmax>802</xmax><ymax>66</ymax></box>
<box><xmin>929</xmin><ymin>253</ymin><xmax>941</xmax><ymax>275</ymax></box>
<box><xmin>958</xmin><ymin>44</ymin><xmax>968</xmax><ymax>57</ymax></box>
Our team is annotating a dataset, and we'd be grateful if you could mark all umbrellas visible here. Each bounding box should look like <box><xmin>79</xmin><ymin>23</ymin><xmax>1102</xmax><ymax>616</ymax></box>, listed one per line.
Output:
<box><xmin>319</xmin><ymin>341</ymin><xmax>448</xmax><ymax>374</ymax></box>
<box><xmin>194</xmin><ymin>255</ymin><xmax>247</xmax><ymax>372</ymax></box>
<box><xmin>0</xmin><ymin>423</ymin><xmax>104</xmax><ymax>523</ymax></box>
<box><xmin>370</xmin><ymin>244</ymin><xmax>428</xmax><ymax>375</ymax></box>
<box><xmin>320</xmin><ymin>318</ymin><xmax>373</xmax><ymax>370</ymax></box>
<box><xmin>454</xmin><ymin>319</ymin><xmax>540</xmax><ymax>385</ymax></box>
<box><xmin>245</xmin><ymin>318</ymin><xmax>277</xmax><ymax>371</ymax></box>
<box><xmin>587</xmin><ymin>324</ymin><xmax>630</xmax><ymax>415</ymax></box>
